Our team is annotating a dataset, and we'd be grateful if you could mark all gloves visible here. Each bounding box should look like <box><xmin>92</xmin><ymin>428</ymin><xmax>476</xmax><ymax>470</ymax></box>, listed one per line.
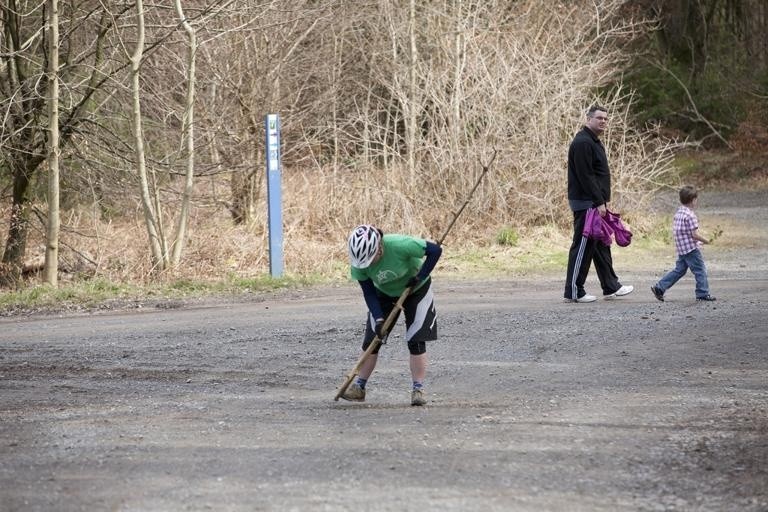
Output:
<box><xmin>374</xmin><ymin>321</ymin><xmax>386</xmax><ymax>337</ymax></box>
<box><xmin>405</xmin><ymin>277</ymin><xmax>418</xmax><ymax>289</ymax></box>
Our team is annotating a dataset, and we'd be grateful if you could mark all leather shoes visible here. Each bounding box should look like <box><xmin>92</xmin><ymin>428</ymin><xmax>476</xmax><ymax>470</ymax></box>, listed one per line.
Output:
<box><xmin>564</xmin><ymin>293</ymin><xmax>597</xmax><ymax>304</ymax></box>
<box><xmin>603</xmin><ymin>285</ymin><xmax>634</xmax><ymax>301</ymax></box>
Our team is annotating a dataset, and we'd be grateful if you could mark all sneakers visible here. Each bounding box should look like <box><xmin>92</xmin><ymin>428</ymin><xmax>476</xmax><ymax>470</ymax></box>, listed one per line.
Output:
<box><xmin>410</xmin><ymin>388</ymin><xmax>427</xmax><ymax>406</ymax></box>
<box><xmin>341</xmin><ymin>383</ymin><xmax>366</xmax><ymax>403</ymax></box>
<box><xmin>696</xmin><ymin>294</ymin><xmax>718</xmax><ymax>302</ymax></box>
<box><xmin>650</xmin><ymin>286</ymin><xmax>664</xmax><ymax>302</ymax></box>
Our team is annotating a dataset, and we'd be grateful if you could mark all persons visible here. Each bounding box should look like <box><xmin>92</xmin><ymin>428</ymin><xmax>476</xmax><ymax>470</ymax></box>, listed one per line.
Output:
<box><xmin>564</xmin><ymin>105</ymin><xmax>634</xmax><ymax>303</ymax></box>
<box><xmin>651</xmin><ymin>186</ymin><xmax>717</xmax><ymax>302</ymax></box>
<box><xmin>341</xmin><ymin>224</ymin><xmax>442</xmax><ymax>406</ymax></box>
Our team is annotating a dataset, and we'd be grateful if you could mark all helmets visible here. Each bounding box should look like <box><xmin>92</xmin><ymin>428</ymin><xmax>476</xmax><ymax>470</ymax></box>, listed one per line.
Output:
<box><xmin>348</xmin><ymin>224</ymin><xmax>381</xmax><ymax>269</ymax></box>
